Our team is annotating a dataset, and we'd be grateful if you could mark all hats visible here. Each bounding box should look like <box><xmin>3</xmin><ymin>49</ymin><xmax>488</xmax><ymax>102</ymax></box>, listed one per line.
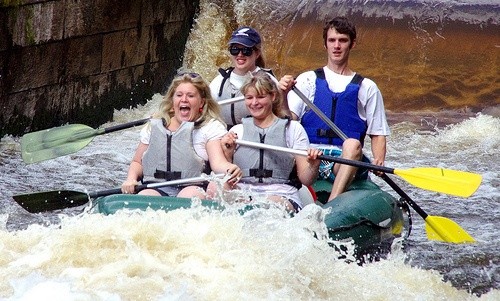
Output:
<box><xmin>228</xmin><ymin>26</ymin><xmax>262</xmax><ymax>48</ymax></box>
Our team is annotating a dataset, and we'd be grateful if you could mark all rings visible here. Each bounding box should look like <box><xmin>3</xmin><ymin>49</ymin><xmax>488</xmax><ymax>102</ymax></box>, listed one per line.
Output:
<box><xmin>124</xmin><ymin>190</ymin><xmax>128</xmax><ymax>194</ymax></box>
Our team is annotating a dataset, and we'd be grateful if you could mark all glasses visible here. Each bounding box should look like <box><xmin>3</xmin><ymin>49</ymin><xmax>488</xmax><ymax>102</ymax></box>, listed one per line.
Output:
<box><xmin>230</xmin><ymin>47</ymin><xmax>252</xmax><ymax>56</ymax></box>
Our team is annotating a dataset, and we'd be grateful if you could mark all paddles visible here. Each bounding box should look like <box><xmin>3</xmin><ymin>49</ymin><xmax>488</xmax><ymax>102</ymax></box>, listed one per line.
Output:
<box><xmin>22</xmin><ymin>96</ymin><xmax>246</xmax><ymax>165</ymax></box>
<box><xmin>286</xmin><ymin>78</ymin><xmax>473</xmax><ymax>243</ymax></box>
<box><xmin>225</xmin><ymin>132</ymin><xmax>483</xmax><ymax>198</ymax></box>
<box><xmin>10</xmin><ymin>170</ymin><xmax>235</xmax><ymax>214</ymax></box>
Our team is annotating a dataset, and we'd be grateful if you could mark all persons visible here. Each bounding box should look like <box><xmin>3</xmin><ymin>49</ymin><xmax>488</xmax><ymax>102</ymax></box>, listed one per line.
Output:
<box><xmin>221</xmin><ymin>72</ymin><xmax>324</xmax><ymax>215</ymax></box>
<box><xmin>208</xmin><ymin>25</ymin><xmax>280</xmax><ymax>131</ymax></box>
<box><xmin>277</xmin><ymin>15</ymin><xmax>390</xmax><ymax>203</ymax></box>
<box><xmin>121</xmin><ymin>72</ymin><xmax>244</xmax><ymax>200</ymax></box>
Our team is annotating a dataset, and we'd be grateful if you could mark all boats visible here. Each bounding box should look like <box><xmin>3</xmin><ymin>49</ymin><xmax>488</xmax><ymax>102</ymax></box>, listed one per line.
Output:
<box><xmin>95</xmin><ymin>176</ymin><xmax>405</xmax><ymax>276</ymax></box>
